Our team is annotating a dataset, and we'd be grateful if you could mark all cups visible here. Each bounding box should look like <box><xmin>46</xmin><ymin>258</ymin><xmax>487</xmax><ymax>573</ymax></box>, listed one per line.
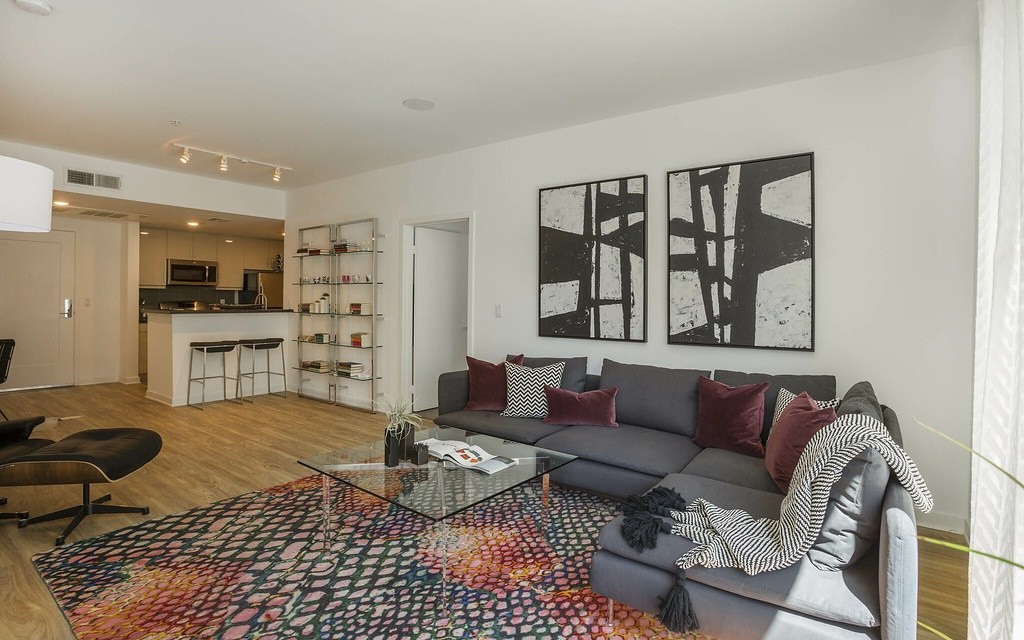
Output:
<box><xmin>310</xmin><ymin>304</ymin><xmax>315</xmax><ymax>313</ymax></box>
<box><xmin>352</xmin><ymin>275</ymin><xmax>360</xmax><ymax>283</ymax></box>
<box><xmin>342</xmin><ymin>275</ymin><xmax>350</xmax><ymax>283</ymax></box>
<box><xmin>301</xmin><ymin>303</ymin><xmax>310</xmax><ymax>312</ymax></box>
<box><xmin>350</xmin><ymin>303</ymin><xmax>361</xmax><ymax>314</ymax></box>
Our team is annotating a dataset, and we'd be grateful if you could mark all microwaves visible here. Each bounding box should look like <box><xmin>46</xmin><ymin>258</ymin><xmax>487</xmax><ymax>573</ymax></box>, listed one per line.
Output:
<box><xmin>168</xmin><ymin>259</ymin><xmax>219</xmax><ymax>285</ymax></box>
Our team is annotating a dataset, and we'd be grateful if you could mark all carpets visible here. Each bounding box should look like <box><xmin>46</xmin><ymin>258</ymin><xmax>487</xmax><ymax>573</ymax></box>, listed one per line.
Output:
<box><xmin>29</xmin><ymin>439</ymin><xmax>725</xmax><ymax>640</ymax></box>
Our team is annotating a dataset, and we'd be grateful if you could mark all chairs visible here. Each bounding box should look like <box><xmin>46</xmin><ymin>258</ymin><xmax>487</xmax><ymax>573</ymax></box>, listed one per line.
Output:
<box><xmin>0</xmin><ymin>415</ymin><xmax>163</xmax><ymax>547</ymax></box>
<box><xmin>0</xmin><ymin>338</ymin><xmax>16</xmax><ymax>421</ymax></box>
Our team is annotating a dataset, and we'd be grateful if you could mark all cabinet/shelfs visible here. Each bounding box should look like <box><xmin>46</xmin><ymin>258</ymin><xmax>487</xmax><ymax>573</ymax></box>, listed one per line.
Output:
<box><xmin>140</xmin><ymin>228</ymin><xmax>283</xmax><ymax>291</ymax></box>
<box><xmin>292</xmin><ymin>216</ymin><xmax>386</xmax><ymax>416</ymax></box>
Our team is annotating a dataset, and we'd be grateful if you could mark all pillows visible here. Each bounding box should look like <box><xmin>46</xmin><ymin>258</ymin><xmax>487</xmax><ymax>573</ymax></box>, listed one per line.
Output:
<box><xmin>521</xmin><ymin>357</ymin><xmax>588</xmax><ymax>394</ymax></box>
<box><xmin>838</xmin><ymin>380</ymin><xmax>885</xmax><ymax>425</ymax></box>
<box><xmin>462</xmin><ymin>353</ymin><xmax>524</xmax><ymax>411</ymax></box>
<box><xmin>713</xmin><ymin>369</ymin><xmax>837</xmax><ymax>448</ymax></box>
<box><xmin>499</xmin><ymin>359</ymin><xmax>566</xmax><ymax>419</ymax></box>
<box><xmin>763</xmin><ymin>391</ymin><xmax>839</xmax><ymax>496</ymax></box>
<box><xmin>598</xmin><ymin>357</ymin><xmax>711</xmax><ymax>437</ymax></box>
<box><xmin>691</xmin><ymin>373</ymin><xmax>772</xmax><ymax>459</ymax></box>
<box><xmin>807</xmin><ymin>446</ymin><xmax>891</xmax><ymax>572</ymax></box>
<box><xmin>765</xmin><ymin>387</ymin><xmax>840</xmax><ymax>451</ymax></box>
<box><xmin>542</xmin><ymin>384</ymin><xmax>622</xmax><ymax>428</ymax></box>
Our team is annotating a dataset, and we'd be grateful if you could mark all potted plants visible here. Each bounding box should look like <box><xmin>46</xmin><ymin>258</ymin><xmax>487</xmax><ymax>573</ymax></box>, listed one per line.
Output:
<box><xmin>369</xmin><ymin>394</ymin><xmax>430</xmax><ymax>468</ymax></box>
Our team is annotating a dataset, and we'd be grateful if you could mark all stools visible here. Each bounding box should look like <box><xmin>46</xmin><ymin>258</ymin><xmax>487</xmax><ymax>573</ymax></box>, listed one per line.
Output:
<box><xmin>186</xmin><ymin>339</ymin><xmax>245</xmax><ymax>411</ymax></box>
<box><xmin>238</xmin><ymin>337</ymin><xmax>289</xmax><ymax>404</ymax></box>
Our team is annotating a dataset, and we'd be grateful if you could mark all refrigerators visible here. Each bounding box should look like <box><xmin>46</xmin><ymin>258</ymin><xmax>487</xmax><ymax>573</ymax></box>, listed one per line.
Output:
<box><xmin>256</xmin><ymin>272</ymin><xmax>283</xmax><ymax>309</ymax></box>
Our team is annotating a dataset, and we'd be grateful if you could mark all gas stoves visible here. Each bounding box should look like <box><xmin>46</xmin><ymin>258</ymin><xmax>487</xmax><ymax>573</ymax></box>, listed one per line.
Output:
<box><xmin>160</xmin><ymin>301</ymin><xmax>195</xmax><ymax>310</ymax></box>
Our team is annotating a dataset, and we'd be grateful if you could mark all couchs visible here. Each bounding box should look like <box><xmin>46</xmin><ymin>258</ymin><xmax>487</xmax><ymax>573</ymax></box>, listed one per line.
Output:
<box><xmin>437</xmin><ymin>352</ymin><xmax>919</xmax><ymax>640</ymax></box>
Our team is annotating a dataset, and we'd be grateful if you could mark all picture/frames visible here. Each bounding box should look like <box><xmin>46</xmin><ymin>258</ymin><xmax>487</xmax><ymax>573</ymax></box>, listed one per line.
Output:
<box><xmin>536</xmin><ymin>174</ymin><xmax>650</xmax><ymax>346</ymax></box>
<box><xmin>666</xmin><ymin>150</ymin><xmax>818</xmax><ymax>353</ymax></box>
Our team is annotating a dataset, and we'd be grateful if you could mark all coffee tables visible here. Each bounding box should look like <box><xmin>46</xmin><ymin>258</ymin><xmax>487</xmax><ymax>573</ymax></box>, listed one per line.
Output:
<box><xmin>294</xmin><ymin>422</ymin><xmax>581</xmax><ymax>620</ymax></box>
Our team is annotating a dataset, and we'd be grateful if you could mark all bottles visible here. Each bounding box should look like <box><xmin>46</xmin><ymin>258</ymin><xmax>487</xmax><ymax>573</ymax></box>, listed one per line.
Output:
<box><xmin>315</xmin><ymin>293</ymin><xmax>329</xmax><ymax>313</ymax></box>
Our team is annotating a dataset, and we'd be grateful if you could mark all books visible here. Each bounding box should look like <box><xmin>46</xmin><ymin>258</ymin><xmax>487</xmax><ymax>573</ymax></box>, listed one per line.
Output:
<box><xmin>298</xmin><ymin>303</ymin><xmax>312</xmax><ymax>312</ymax></box>
<box><xmin>351</xmin><ymin>332</ymin><xmax>371</xmax><ymax>347</ymax></box>
<box><xmin>334</xmin><ymin>243</ymin><xmax>358</xmax><ymax>253</ymax></box>
<box><xmin>297</xmin><ymin>248</ymin><xmax>330</xmax><ymax>256</ymax></box>
<box><xmin>302</xmin><ymin>360</ymin><xmax>329</xmax><ymax>373</ymax></box>
<box><xmin>414</xmin><ymin>438</ymin><xmax>516</xmax><ymax>474</ymax></box>
<box><xmin>338</xmin><ymin>362</ymin><xmax>363</xmax><ymax>378</ymax></box>
<box><xmin>350</xmin><ymin>303</ymin><xmax>370</xmax><ymax>315</ymax></box>
<box><xmin>315</xmin><ymin>333</ymin><xmax>330</xmax><ymax>343</ymax></box>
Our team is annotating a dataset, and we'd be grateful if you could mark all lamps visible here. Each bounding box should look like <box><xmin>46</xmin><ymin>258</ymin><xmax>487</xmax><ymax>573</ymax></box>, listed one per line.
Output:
<box><xmin>179</xmin><ymin>147</ymin><xmax>193</xmax><ymax>165</ymax></box>
<box><xmin>272</xmin><ymin>166</ymin><xmax>284</xmax><ymax>183</ymax></box>
<box><xmin>218</xmin><ymin>155</ymin><xmax>230</xmax><ymax>173</ymax></box>
<box><xmin>0</xmin><ymin>155</ymin><xmax>55</xmax><ymax>234</ymax></box>
